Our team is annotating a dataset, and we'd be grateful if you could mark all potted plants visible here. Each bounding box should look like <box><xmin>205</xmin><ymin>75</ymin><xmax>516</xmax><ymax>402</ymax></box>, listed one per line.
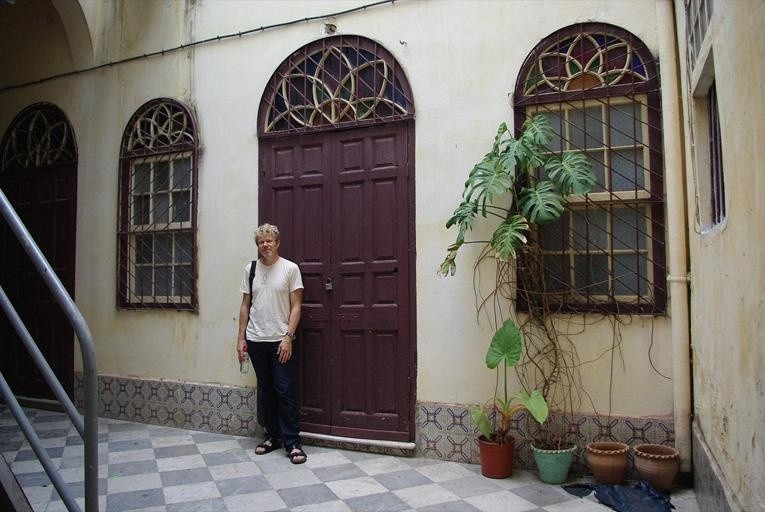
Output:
<box><xmin>466</xmin><ymin>317</ymin><xmax>549</xmax><ymax>480</ymax></box>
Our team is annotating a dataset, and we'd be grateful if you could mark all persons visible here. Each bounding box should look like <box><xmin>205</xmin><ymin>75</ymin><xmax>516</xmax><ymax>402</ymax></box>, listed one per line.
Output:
<box><xmin>234</xmin><ymin>221</ymin><xmax>310</xmax><ymax>466</ymax></box>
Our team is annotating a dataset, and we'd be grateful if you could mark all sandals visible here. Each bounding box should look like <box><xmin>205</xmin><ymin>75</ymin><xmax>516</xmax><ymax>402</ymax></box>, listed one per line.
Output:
<box><xmin>255</xmin><ymin>437</ymin><xmax>282</xmax><ymax>455</ymax></box>
<box><xmin>288</xmin><ymin>447</ymin><xmax>307</xmax><ymax>464</ymax></box>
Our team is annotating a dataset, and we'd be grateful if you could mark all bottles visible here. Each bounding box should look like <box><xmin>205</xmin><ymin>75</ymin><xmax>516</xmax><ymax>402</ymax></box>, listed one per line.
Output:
<box><xmin>240</xmin><ymin>347</ymin><xmax>249</xmax><ymax>374</ymax></box>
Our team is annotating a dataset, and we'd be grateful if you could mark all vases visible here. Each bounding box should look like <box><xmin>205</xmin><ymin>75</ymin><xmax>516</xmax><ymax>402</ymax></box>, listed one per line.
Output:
<box><xmin>530</xmin><ymin>438</ymin><xmax>680</xmax><ymax>493</ymax></box>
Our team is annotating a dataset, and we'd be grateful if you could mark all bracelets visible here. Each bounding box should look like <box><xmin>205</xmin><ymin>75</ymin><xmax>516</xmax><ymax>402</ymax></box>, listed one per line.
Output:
<box><xmin>286</xmin><ymin>330</ymin><xmax>297</xmax><ymax>342</ymax></box>
